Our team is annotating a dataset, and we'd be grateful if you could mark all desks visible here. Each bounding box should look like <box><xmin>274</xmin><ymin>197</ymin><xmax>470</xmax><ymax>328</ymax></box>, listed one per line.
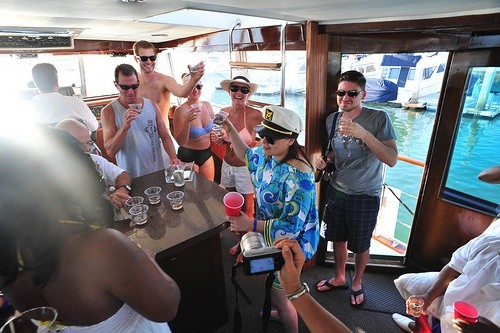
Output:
<box><xmin>111</xmin><ymin>169</ymin><xmax>232</xmax><ymax>333</ymax></box>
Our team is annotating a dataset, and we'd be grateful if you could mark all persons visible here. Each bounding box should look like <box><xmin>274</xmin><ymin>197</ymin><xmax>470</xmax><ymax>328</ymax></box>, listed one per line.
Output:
<box><xmin>391</xmin><ymin>167</ymin><xmax>500</xmax><ymax>333</ymax></box>
<box><xmin>313</xmin><ymin>70</ymin><xmax>398</xmax><ymax>305</ymax></box>
<box><xmin>272</xmin><ymin>237</ymin><xmax>353</xmax><ymax>333</ymax></box>
<box><xmin>0</xmin><ymin>126</ymin><xmax>181</xmax><ymax>333</ymax></box>
<box><xmin>133</xmin><ymin>40</ymin><xmax>205</xmax><ymax>164</ymax></box>
<box><xmin>54</xmin><ymin>119</ymin><xmax>131</xmax><ymax>208</ymax></box>
<box><xmin>23</xmin><ymin>63</ymin><xmax>98</xmax><ymax>137</ymax></box>
<box><xmin>173</xmin><ymin>73</ymin><xmax>214</xmax><ymax>182</ymax></box>
<box><xmin>215</xmin><ymin>105</ymin><xmax>320</xmax><ymax>333</ymax></box>
<box><xmin>210</xmin><ymin>76</ymin><xmax>263</xmax><ymax>263</ymax></box>
<box><xmin>101</xmin><ymin>63</ymin><xmax>184</xmax><ymax>179</ymax></box>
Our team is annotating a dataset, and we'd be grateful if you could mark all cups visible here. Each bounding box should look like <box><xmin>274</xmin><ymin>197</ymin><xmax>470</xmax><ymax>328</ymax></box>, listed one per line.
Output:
<box><xmin>144</xmin><ymin>186</ymin><xmax>161</xmax><ymax>204</ymax></box>
<box><xmin>166</xmin><ymin>191</ymin><xmax>184</xmax><ymax>210</ymax></box>
<box><xmin>190</xmin><ymin>103</ymin><xmax>202</xmax><ymax>115</ymax></box>
<box><xmin>212</xmin><ymin>108</ymin><xmax>229</xmax><ymax>128</ymax></box>
<box><xmin>212</xmin><ymin>127</ymin><xmax>222</xmax><ymax>144</ymax></box>
<box><xmin>0</xmin><ymin>305</ymin><xmax>58</xmax><ymax>333</ymax></box>
<box><xmin>172</xmin><ymin>165</ymin><xmax>185</xmax><ymax>187</ymax></box>
<box><xmin>128</xmin><ymin>103</ymin><xmax>142</xmax><ymax>119</ymax></box>
<box><xmin>125</xmin><ymin>196</ymin><xmax>149</xmax><ymax>225</ymax></box>
<box><xmin>409</xmin><ymin>295</ymin><xmax>424</xmax><ymax>318</ymax></box>
<box><xmin>453</xmin><ymin>299</ymin><xmax>479</xmax><ymax>323</ymax></box>
<box><xmin>223</xmin><ymin>192</ymin><xmax>245</xmax><ymax>217</ymax></box>
<box><xmin>339</xmin><ymin>118</ymin><xmax>352</xmax><ymax>143</ymax></box>
<box><xmin>189</xmin><ymin>59</ymin><xmax>201</xmax><ymax>73</ymax></box>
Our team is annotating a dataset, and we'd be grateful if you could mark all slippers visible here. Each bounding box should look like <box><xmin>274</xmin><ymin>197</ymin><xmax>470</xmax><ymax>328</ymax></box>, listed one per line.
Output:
<box><xmin>314</xmin><ymin>279</ymin><xmax>349</xmax><ymax>293</ymax></box>
<box><xmin>350</xmin><ymin>283</ymin><xmax>365</xmax><ymax>309</ymax></box>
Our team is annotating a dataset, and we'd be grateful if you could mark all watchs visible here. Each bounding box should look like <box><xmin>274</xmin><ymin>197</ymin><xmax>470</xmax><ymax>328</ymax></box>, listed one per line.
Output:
<box><xmin>121</xmin><ymin>185</ymin><xmax>131</xmax><ymax>191</ymax></box>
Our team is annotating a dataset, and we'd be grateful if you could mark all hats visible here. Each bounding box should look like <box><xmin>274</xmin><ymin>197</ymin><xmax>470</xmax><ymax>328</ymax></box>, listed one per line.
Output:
<box><xmin>253</xmin><ymin>104</ymin><xmax>302</xmax><ymax>139</ymax></box>
<box><xmin>478</xmin><ymin>166</ymin><xmax>500</xmax><ymax>184</ymax></box>
<box><xmin>220</xmin><ymin>75</ymin><xmax>258</xmax><ymax>99</ymax></box>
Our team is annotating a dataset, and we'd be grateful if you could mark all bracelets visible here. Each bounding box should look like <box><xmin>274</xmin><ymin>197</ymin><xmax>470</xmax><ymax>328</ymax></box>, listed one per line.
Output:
<box><xmin>252</xmin><ymin>219</ymin><xmax>257</xmax><ymax>232</ymax></box>
<box><xmin>288</xmin><ymin>282</ymin><xmax>310</xmax><ymax>301</ymax></box>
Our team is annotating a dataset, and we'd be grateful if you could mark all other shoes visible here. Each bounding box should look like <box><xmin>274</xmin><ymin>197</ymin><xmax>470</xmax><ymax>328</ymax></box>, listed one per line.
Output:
<box><xmin>392</xmin><ymin>312</ymin><xmax>416</xmax><ymax>333</ymax></box>
<box><xmin>260</xmin><ymin>308</ymin><xmax>282</xmax><ymax>322</ymax></box>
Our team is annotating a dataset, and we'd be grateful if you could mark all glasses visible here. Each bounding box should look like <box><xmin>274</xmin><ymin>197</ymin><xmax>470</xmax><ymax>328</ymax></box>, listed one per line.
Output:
<box><xmin>259</xmin><ymin>132</ymin><xmax>284</xmax><ymax>145</ymax></box>
<box><xmin>229</xmin><ymin>85</ymin><xmax>251</xmax><ymax>94</ymax></box>
<box><xmin>118</xmin><ymin>80</ymin><xmax>139</xmax><ymax>90</ymax></box>
<box><xmin>196</xmin><ymin>85</ymin><xmax>203</xmax><ymax>90</ymax></box>
<box><xmin>335</xmin><ymin>88</ymin><xmax>364</xmax><ymax>98</ymax></box>
<box><xmin>136</xmin><ymin>55</ymin><xmax>156</xmax><ymax>62</ymax></box>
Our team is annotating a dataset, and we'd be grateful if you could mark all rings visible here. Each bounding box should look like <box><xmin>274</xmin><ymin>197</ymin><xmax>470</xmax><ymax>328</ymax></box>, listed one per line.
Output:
<box><xmin>349</xmin><ymin>129</ymin><xmax>351</xmax><ymax>132</ymax></box>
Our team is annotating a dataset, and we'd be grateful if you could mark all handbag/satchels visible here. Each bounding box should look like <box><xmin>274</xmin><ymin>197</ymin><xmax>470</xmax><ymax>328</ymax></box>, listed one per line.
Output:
<box><xmin>315</xmin><ymin>162</ymin><xmax>336</xmax><ymax>185</ymax></box>
